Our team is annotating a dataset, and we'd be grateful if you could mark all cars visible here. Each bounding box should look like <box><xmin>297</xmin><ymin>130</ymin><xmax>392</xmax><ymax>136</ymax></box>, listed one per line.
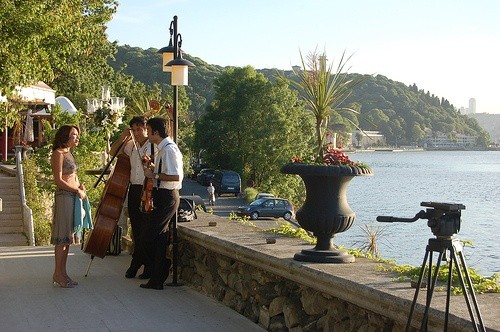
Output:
<box><xmin>255</xmin><ymin>193</ymin><xmax>274</xmax><ymax>200</ymax></box>
<box><xmin>192</xmin><ymin>163</ymin><xmax>203</xmax><ymax>172</ymax></box>
<box><xmin>237</xmin><ymin>198</ymin><xmax>294</xmax><ymax>221</ymax></box>
<box><xmin>197</xmin><ymin>168</ymin><xmax>213</xmax><ymax>186</ymax></box>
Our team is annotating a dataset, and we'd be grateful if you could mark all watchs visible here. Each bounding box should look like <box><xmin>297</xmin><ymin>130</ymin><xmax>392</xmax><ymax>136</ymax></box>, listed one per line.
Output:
<box><xmin>155</xmin><ymin>174</ymin><xmax>159</xmax><ymax>179</ymax></box>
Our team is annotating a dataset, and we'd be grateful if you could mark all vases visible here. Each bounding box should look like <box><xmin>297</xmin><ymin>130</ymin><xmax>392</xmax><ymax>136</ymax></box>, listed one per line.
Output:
<box><xmin>281</xmin><ymin>164</ymin><xmax>373</xmax><ymax>264</ymax></box>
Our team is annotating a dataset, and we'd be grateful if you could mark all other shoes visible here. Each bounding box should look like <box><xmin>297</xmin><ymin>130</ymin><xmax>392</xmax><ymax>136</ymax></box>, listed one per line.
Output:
<box><xmin>138</xmin><ymin>271</ymin><xmax>151</xmax><ymax>279</ymax></box>
<box><xmin>140</xmin><ymin>281</ymin><xmax>163</xmax><ymax>290</ymax></box>
<box><xmin>125</xmin><ymin>265</ymin><xmax>138</xmax><ymax>278</ymax></box>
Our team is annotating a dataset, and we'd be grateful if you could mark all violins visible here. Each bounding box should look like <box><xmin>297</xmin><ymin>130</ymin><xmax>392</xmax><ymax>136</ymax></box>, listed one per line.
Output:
<box><xmin>139</xmin><ymin>154</ymin><xmax>154</xmax><ymax>214</ymax></box>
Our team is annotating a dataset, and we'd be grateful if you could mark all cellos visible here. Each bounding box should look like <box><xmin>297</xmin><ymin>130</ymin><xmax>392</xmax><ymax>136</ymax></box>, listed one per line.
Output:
<box><xmin>83</xmin><ymin>129</ymin><xmax>131</xmax><ymax>277</ymax></box>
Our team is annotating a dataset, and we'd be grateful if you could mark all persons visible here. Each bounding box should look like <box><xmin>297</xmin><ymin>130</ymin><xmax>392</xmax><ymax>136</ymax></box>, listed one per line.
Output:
<box><xmin>109</xmin><ymin>116</ymin><xmax>159</xmax><ymax>280</ymax></box>
<box><xmin>51</xmin><ymin>125</ymin><xmax>87</xmax><ymax>288</ymax></box>
<box><xmin>207</xmin><ymin>183</ymin><xmax>215</xmax><ymax>206</ymax></box>
<box><xmin>140</xmin><ymin>117</ymin><xmax>184</xmax><ymax>289</ymax></box>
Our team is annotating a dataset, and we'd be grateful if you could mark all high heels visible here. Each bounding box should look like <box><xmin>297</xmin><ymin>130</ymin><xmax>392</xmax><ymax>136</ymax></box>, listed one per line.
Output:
<box><xmin>52</xmin><ymin>275</ymin><xmax>79</xmax><ymax>288</ymax></box>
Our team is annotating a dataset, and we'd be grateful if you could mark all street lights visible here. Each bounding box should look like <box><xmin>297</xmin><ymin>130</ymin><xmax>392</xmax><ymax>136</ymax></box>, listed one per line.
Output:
<box><xmin>157</xmin><ymin>15</ymin><xmax>195</xmax><ymax>286</ymax></box>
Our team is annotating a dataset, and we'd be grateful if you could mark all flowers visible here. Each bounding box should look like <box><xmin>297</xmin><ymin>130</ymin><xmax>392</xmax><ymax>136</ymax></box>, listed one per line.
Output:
<box><xmin>274</xmin><ymin>46</ymin><xmax>379</xmax><ymax>165</ymax></box>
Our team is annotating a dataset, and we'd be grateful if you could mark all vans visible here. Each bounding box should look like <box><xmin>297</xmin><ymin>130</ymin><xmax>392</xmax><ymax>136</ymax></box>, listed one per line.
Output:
<box><xmin>212</xmin><ymin>169</ymin><xmax>241</xmax><ymax>196</ymax></box>
